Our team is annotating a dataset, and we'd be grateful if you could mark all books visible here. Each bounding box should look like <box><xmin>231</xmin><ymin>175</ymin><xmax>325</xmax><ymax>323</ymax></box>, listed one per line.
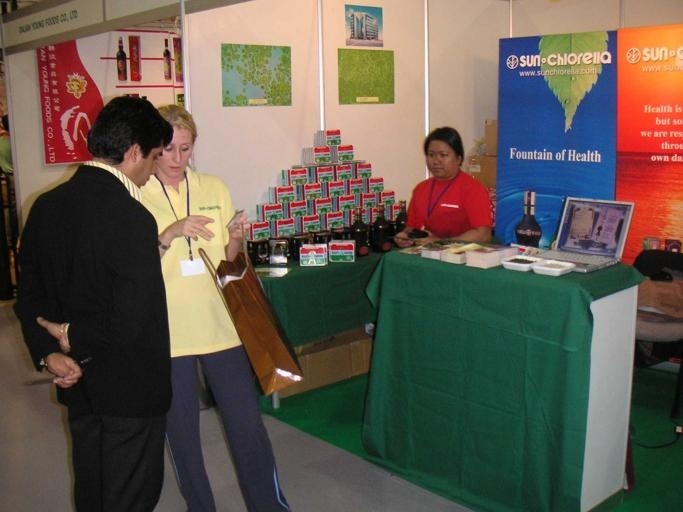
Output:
<box><xmin>421</xmin><ymin>241</ymin><xmax>518</xmax><ymax>270</ymax></box>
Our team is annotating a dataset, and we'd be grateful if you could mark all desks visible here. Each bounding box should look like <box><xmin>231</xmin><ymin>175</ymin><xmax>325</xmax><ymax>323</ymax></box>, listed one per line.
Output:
<box><xmin>360</xmin><ymin>240</ymin><xmax>647</xmax><ymax>512</ymax></box>
<box><xmin>252</xmin><ymin>240</ymin><xmax>416</xmax><ymax>408</ymax></box>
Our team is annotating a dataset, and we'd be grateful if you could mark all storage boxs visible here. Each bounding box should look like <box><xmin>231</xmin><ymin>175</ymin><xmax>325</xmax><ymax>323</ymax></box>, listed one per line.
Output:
<box><xmin>342</xmin><ymin>323</ymin><xmax>374</xmax><ymax>376</ymax></box>
<box><xmin>277</xmin><ymin>333</ymin><xmax>351</xmax><ymax>402</ymax></box>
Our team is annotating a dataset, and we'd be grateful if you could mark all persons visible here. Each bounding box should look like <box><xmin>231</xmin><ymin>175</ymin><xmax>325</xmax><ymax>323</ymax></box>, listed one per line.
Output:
<box><xmin>12</xmin><ymin>96</ymin><xmax>175</xmax><ymax>512</ymax></box>
<box><xmin>393</xmin><ymin>127</ymin><xmax>495</xmax><ymax>249</ymax></box>
<box><xmin>140</xmin><ymin>105</ymin><xmax>293</xmax><ymax>512</ymax></box>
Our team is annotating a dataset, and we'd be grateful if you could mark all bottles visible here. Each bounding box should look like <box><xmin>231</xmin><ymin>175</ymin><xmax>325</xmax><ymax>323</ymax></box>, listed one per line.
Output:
<box><xmin>395</xmin><ymin>200</ymin><xmax>408</xmax><ymax>232</ymax></box>
<box><xmin>116</xmin><ymin>37</ymin><xmax>127</xmax><ymax>81</ymax></box>
<box><xmin>514</xmin><ymin>190</ymin><xmax>542</xmax><ymax>249</ymax></box>
<box><xmin>350</xmin><ymin>206</ymin><xmax>369</xmax><ymax>257</ymax></box>
<box><xmin>162</xmin><ymin>38</ymin><xmax>171</xmax><ymax>81</ymax></box>
<box><xmin>371</xmin><ymin>203</ymin><xmax>391</xmax><ymax>254</ymax></box>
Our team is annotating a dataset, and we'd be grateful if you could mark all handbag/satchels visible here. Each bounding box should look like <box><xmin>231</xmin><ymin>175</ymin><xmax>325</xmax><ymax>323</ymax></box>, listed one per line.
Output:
<box><xmin>197</xmin><ymin>221</ymin><xmax>305</xmax><ymax>396</ymax></box>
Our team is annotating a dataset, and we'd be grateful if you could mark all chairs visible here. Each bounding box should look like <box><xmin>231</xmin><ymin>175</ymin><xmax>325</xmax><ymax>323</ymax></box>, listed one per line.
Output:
<box><xmin>629</xmin><ymin>249</ymin><xmax>683</xmax><ymax>421</ymax></box>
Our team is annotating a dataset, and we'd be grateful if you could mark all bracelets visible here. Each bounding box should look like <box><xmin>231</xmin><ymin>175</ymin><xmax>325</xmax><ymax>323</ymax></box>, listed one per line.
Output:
<box><xmin>156</xmin><ymin>237</ymin><xmax>171</xmax><ymax>250</ymax></box>
<box><xmin>42</xmin><ymin>356</ymin><xmax>48</xmax><ymax>368</ymax></box>
<box><xmin>59</xmin><ymin>322</ymin><xmax>69</xmax><ymax>349</ymax></box>
